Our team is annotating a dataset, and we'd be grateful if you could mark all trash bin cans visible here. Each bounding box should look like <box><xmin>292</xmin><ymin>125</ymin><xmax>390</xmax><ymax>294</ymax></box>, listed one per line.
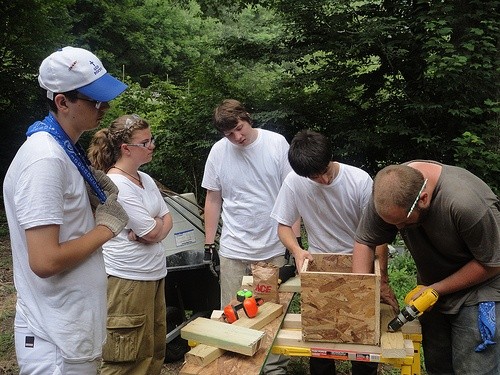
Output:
<box><xmin>164</xmin><ymin>249</ymin><xmax>211</xmax><ymax>363</ymax></box>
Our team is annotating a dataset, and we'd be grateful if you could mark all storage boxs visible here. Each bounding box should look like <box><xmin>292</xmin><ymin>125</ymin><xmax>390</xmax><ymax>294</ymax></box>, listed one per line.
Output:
<box><xmin>159</xmin><ymin>193</ymin><xmax>207</xmax><ymax>254</ymax></box>
<box><xmin>299</xmin><ymin>253</ymin><xmax>383</xmax><ymax>345</ymax></box>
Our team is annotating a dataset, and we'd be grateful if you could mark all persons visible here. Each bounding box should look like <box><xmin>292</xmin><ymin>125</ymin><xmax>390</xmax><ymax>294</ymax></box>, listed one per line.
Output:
<box><xmin>2</xmin><ymin>46</ymin><xmax>128</xmax><ymax>375</ymax></box>
<box><xmin>87</xmin><ymin>115</ymin><xmax>174</xmax><ymax>375</ymax></box>
<box><xmin>352</xmin><ymin>160</ymin><xmax>500</xmax><ymax>375</ymax></box>
<box><xmin>269</xmin><ymin>129</ymin><xmax>401</xmax><ymax>375</ymax></box>
<box><xmin>200</xmin><ymin>99</ymin><xmax>302</xmax><ymax>313</ymax></box>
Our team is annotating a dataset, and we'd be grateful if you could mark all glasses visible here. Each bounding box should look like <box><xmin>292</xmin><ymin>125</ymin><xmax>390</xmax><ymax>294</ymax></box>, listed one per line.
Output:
<box><xmin>119</xmin><ymin>139</ymin><xmax>156</xmax><ymax>149</ymax></box>
<box><xmin>69</xmin><ymin>97</ymin><xmax>103</xmax><ymax>109</ymax></box>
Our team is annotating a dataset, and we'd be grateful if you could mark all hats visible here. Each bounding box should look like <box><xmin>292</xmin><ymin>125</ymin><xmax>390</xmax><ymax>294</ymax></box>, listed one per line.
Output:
<box><xmin>38</xmin><ymin>46</ymin><xmax>128</xmax><ymax>102</ymax></box>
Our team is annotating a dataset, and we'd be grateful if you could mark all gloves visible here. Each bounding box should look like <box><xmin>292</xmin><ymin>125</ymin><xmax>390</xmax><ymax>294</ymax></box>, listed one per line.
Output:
<box><xmin>95</xmin><ymin>191</ymin><xmax>129</xmax><ymax>237</ymax></box>
<box><xmin>203</xmin><ymin>243</ymin><xmax>220</xmax><ymax>282</ymax></box>
<box><xmin>85</xmin><ymin>165</ymin><xmax>119</xmax><ymax>213</ymax></box>
<box><xmin>285</xmin><ymin>237</ymin><xmax>304</xmax><ymax>274</ymax></box>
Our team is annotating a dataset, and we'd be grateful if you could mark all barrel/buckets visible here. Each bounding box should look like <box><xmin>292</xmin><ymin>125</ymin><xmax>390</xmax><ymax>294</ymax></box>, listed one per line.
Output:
<box><xmin>163</xmin><ymin>250</ymin><xmax>223</xmax><ymax>364</ymax></box>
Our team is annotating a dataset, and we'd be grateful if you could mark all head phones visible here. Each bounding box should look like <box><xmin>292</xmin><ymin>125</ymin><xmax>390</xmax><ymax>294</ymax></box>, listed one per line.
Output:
<box><xmin>221</xmin><ymin>296</ymin><xmax>264</xmax><ymax>323</ymax></box>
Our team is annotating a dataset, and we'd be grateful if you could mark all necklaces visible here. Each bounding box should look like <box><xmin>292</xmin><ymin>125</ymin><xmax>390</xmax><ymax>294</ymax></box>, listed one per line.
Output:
<box><xmin>106</xmin><ymin>165</ymin><xmax>142</xmax><ymax>185</ymax></box>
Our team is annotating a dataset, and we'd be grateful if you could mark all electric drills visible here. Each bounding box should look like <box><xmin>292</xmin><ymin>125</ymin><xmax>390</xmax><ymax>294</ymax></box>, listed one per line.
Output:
<box><xmin>386</xmin><ymin>285</ymin><xmax>440</xmax><ymax>333</ymax></box>
